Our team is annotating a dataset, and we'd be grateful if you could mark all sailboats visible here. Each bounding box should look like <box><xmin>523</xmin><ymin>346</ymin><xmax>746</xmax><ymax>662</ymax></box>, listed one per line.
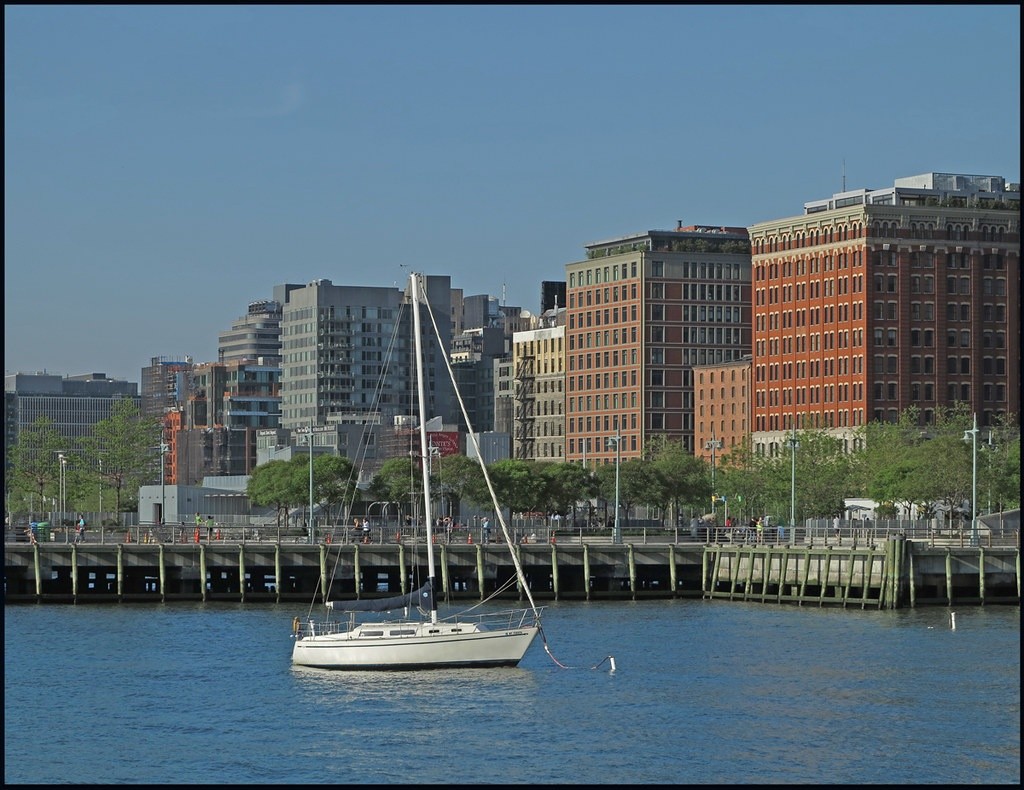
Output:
<box><xmin>292</xmin><ymin>270</ymin><xmax>549</xmax><ymax>670</ymax></box>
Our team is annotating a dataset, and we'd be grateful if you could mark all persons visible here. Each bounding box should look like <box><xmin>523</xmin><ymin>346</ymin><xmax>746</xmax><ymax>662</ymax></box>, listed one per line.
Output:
<box><xmin>350</xmin><ymin>519</ymin><xmax>373</xmax><ymax>544</ymax></box>
<box><xmin>195</xmin><ymin>512</ymin><xmax>213</xmax><ymax>539</ymax></box>
<box><xmin>24</xmin><ymin>514</ymin><xmax>87</xmax><ymax>543</ymax></box>
<box><xmin>406</xmin><ymin>514</ymin><xmax>490</xmax><ymax>545</ymax></box>
<box><xmin>832</xmin><ymin>516</ymin><xmax>841</xmax><ymax>543</ymax></box>
<box><xmin>679</xmin><ymin>513</ymin><xmax>771</xmax><ymax>544</ymax></box>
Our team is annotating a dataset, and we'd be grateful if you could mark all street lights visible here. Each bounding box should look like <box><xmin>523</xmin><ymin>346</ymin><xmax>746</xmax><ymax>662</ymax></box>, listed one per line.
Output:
<box><xmin>605</xmin><ymin>421</ymin><xmax>624</xmax><ymax>544</ymax></box>
<box><xmin>158</xmin><ymin>430</ymin><xmax>175</xmax><ymax>523</ymax></box>
<box><xmin>53</xmin><ymin>449</ymin><xmax>69</xmax><ymax>527</ymax></box>
<box><xmin>60</xmin><ymin>455</ymin><xmax>72</xmax><ymax>524</ymax></box>
<box><xmin>787</xmin><ymin>424</ymin><xmax>802</xmax><ymax>544</ymax></box>
<box><xmin>298</xmin><ymin>416</ymin><xmax>318</xmax><ymax>544</ymax></box>
<box><xmin>958</xmin><ymin>412</ymin><xmax>980</xmax><ymax>546</ymax></box>
<box><xmin>703</xmin><ymin>431</ymin><xmax>726</xmax><ymax>515</ymax></box>
<box><xmin>429</xmin><ymin>434</ymin><xmax>442</xmax><ymax>477</ymax></box>
